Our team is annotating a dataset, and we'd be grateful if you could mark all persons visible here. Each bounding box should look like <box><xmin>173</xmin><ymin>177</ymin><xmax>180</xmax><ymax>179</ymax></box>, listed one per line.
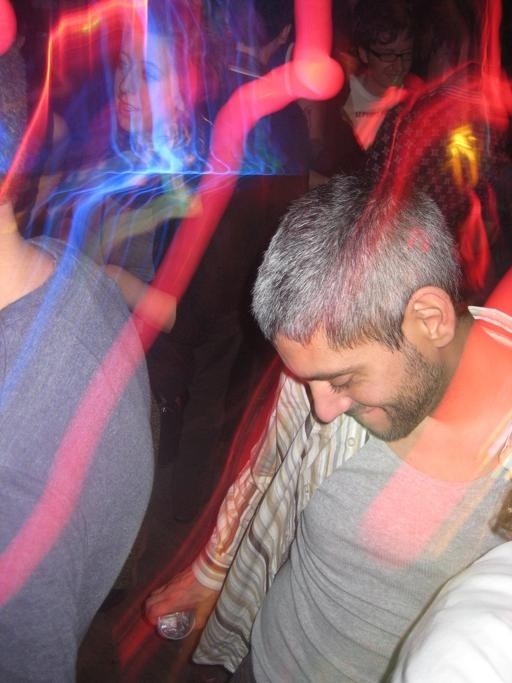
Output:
<box><xmin>15</xmin><ymin>2</ymin><xmax>512</xmax><ymax>527</ymax></box>
<box><xmin>1</xmin><ymin>43</ymin><xmax>159</xmax><ymax>683</ymax></box>
<box><xmin>142</xmin><ymin>169</ymin><xmax>512</xmax><ymax>681</ymax></box>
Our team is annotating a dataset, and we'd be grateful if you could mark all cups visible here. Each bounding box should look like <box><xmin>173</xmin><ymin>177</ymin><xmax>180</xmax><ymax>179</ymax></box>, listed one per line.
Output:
<box><xmin>157</xmin><ymin>609</ymin><xmax>196</xmax><ymax>640</ymax></box>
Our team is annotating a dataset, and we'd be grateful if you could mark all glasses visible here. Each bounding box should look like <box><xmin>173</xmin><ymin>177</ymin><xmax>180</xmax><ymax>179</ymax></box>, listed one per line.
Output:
<box><xmin>366</xmin><ymin>43</ymin><xmax>418</xmax><ymax>65</ymax></box>
